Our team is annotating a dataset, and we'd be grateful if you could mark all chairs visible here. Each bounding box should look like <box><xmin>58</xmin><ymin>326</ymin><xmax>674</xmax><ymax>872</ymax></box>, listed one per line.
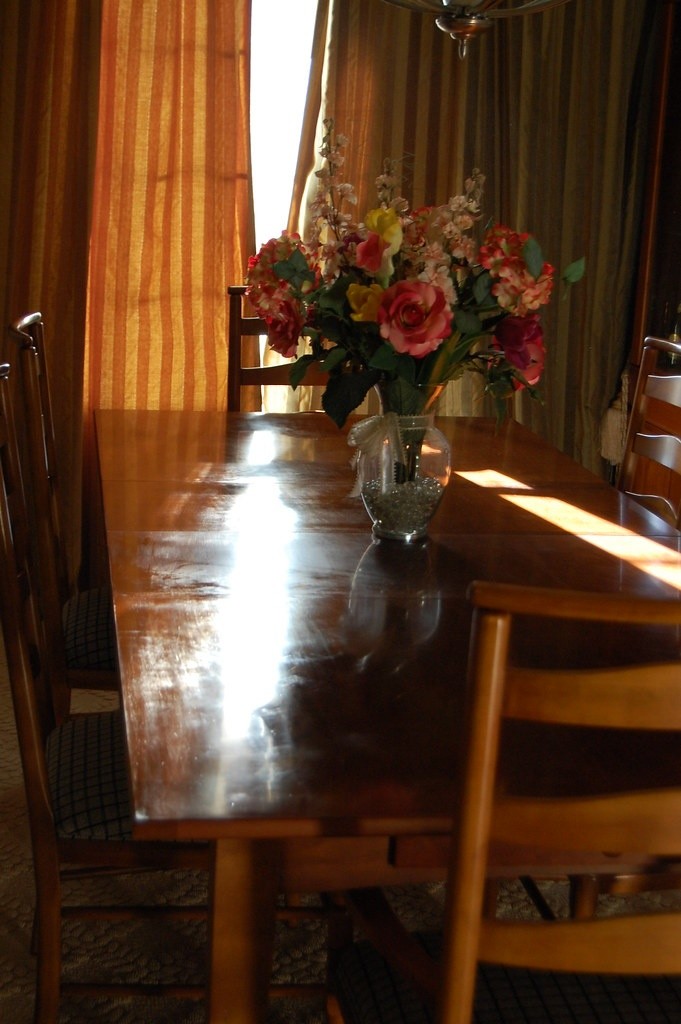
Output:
<box><xmin>614</xmin><ymin>335</ymin><xmax>681</xmax><ymax>534</ymax></box>
<box><xmin>0</xmin><ymin>363</ymin><xmax>355</xmax><ymax>1024</ymax></box>
<box><xmin>328</xmin><ymin>579</ymin><xmax>681</xmax><ymax>1024</ymax></box>
<box><xmin>224</xmin><ymin>284</ymin><xmax>352</xmax><ymax>412</ymax></box>
<box><xmin>0</xmin><ymin>311</ymin><xmax>120</xmax><ymax>724</ymax></box>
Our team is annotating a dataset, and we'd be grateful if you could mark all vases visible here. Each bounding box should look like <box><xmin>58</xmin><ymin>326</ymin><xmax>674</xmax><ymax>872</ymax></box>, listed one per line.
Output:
<box><xmin>346</xmin><ymin>382</ymin><xmax>460</xmax><ymax>544</ymax></box>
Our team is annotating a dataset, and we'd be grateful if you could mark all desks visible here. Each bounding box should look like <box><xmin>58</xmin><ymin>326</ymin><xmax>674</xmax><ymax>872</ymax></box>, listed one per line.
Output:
<box><xmin>93</xmin><ymin>409</ymin><xmax>681</xmax><ymax>1024</ymax></box>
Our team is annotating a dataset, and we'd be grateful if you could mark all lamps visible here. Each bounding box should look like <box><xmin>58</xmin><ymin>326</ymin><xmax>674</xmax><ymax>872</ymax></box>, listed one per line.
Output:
<box><xmin>435</xmin><ymin>14</ymin><xmax>495</xmax><ymax>64</ymax></box>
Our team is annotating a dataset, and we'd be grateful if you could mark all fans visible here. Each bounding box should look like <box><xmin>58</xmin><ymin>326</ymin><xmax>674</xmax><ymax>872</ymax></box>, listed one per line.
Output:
<box><xmin>378</xmin><ymin>0</ymin><xmax>570</xmax><ymax>19</ymax></box>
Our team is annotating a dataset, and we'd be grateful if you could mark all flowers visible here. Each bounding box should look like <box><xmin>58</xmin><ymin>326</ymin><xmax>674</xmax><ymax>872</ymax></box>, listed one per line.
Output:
<box><xmin>238</xmin><ymin>108</ymin><xmax>589</xmax><ymax>483</ymax></box>
